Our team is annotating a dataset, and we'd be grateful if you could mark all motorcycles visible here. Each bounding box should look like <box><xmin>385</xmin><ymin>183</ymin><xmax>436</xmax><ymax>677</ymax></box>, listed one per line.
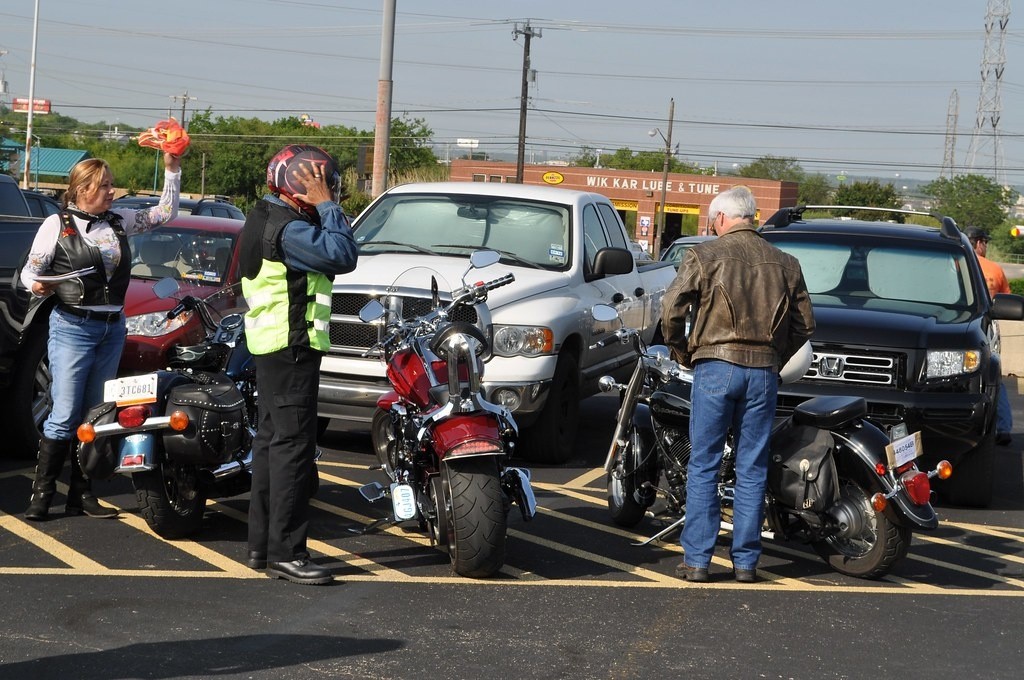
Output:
<box><xmin>587</xmin><ymin>301</ymin><xmax>959</xmax><ymax>579</ymax></box>
<box><xmin>77</xmin><ymin>276</ymin><xmax>324</xmax><ymax>541</ymax></box>
<box><xmin>346</xmin><ymin>250</ymin><xmax>538</xmax><ymax>579</ymax></box>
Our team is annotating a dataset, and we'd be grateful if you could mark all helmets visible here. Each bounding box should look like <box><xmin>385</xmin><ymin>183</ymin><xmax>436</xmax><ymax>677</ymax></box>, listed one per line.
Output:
<box><xmin>267</xmin><ymin>143</ymin><xmax>343</xmax><ymax>227</ymax></box>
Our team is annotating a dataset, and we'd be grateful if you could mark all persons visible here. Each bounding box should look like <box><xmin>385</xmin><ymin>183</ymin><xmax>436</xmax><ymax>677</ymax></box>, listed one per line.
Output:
<box><xmin>954</xmin><ymin>226</ymin><xmax>1012</xmax><ymax>302</ymax></box>
<box><xmin>661</xmin><ymin>186</ymin><xmax>817</xmax><ymax>583</ymax></box>
<box><xmin>21</xmin><ymin>150</ymin><xmax>183</xmax><ymax>521</ymax></box>
<box><xmin>237</xmin><ymin>144</ymin><xmax>359</xmax><ymax>585</ymax></box>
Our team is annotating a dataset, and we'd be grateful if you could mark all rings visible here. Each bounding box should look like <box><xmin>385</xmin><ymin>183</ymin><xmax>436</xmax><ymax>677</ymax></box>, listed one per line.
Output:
<box><xmin>315</xmin><ymin>174</ymin><xmax>321</xmax><ymax>178</ymax></box>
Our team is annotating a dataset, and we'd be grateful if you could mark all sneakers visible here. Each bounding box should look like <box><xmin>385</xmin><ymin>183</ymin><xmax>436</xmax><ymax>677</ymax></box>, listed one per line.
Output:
<box><xmin>675</xmin><ymin>561</ymin><xmax>708</xmax><ymax>581</ymax></box>
<box><xmin>732</xmin><ymin>566</ymin><xmax>756</xmax><ymax>581</ymax></box>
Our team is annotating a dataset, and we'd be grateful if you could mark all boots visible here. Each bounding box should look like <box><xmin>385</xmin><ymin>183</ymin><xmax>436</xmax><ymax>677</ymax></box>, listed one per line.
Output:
<box><xmin>23</xmin><ymin>432</ymin><xmax>71</xmax><ymax>520</ymax></box>
<box><xmin>65</xmin><ymin>435</ymin><xmax>119</xmax><ymax>518</ymax></box>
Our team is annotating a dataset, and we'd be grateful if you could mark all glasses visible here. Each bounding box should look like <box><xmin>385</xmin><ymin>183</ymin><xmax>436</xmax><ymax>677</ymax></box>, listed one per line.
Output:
<box><xmin>711</xmin><ymin>211</ymin><xmax>725</xmax><ymax>235</ymax></box>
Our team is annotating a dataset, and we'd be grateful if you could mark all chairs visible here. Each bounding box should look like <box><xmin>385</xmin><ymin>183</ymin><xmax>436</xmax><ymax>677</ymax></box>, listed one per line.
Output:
<box><xmin>130</xmin><ymin>241</ymin><xmax>181</xmax><ymax>277</ymax></box>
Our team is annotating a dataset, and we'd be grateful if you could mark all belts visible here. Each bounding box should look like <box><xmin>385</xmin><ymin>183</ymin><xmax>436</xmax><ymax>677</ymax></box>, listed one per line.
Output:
<box><xmin>55</xmin><ymin>303</ymin><xmax>126</xmax><ymax>324</ymax></box>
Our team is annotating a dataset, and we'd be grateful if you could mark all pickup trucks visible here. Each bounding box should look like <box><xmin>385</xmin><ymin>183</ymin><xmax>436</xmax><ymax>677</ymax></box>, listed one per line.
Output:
<box><xmin>317</xmin><ymin>179</ymin><xmax>678</xmax><ymax>445</ymax></box>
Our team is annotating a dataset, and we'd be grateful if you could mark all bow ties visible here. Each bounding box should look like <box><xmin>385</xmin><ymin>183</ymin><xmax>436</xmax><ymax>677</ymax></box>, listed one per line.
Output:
<box><xmin>66</xmin><ymin>207</ymin><xmax>113</xmax><ymax>233</ymax></box>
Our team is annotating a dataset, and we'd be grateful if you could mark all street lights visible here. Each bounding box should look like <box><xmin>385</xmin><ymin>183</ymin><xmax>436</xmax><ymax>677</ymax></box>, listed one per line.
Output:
<box><xmin>647</xmin><ymin>127</ymin><xmax>671</xmax><ymax>261</ymax></box>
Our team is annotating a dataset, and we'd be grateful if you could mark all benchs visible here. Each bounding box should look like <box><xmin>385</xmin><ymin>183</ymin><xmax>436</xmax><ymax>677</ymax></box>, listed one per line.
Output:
<box><xmin>167</xmin><ymin>246</ymin><xmax>230</xmax><ymax>277</ymax></box>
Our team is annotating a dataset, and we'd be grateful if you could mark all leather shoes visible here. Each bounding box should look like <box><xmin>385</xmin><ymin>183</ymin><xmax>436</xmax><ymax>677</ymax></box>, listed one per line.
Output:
<box><xmin>248</xmin><ymin>548</ymin><xmax>268</xmax><ymax>569</ymax></box>
<box><xmin>268</xmin><ymin>554</ymin><xmax>333</xmax><ymax>583</ymax></box>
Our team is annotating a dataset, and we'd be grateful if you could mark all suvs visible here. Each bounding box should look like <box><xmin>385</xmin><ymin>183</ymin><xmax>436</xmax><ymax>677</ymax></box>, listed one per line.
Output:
<box><xmin>0</xmin><ymin>173</ymin><xmax>246</xmax><ymax>469</ymax></box>
<box><xmin>757</xmin><ymin>200</ymin><xmax>1024</xmax><ymax>505</ymax></box>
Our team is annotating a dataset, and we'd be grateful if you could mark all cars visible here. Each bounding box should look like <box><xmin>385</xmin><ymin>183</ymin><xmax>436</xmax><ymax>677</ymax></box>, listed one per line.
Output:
<box><xmin>659</xmin><ymin>234</ymin><xmax>720</xmax><ymax>272</ymax></box>
<box><xmin>631</xmin><ymin>242</ymin><xmax>655</xmax><ymax>261</ymax></box>
